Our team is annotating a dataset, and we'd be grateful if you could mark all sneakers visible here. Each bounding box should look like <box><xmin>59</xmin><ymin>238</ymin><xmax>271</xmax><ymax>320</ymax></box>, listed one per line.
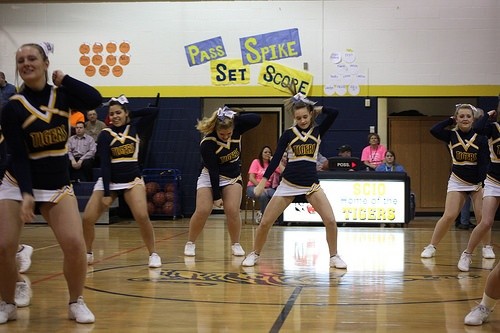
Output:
<box><xmin>87</xmin><ymin>252</ymin><xmax>94</xmax><ymax>264</ymax></box>
<box><xmin>15</xmin><ymin>280</ymin><xmax>30</xmax><ymax>306</ymax></box>
<box><xmin>255</xmin><ymin>210</ymin><xmax>262</xmax><ymax>224</ymax></box>
<box><xmin>86</xmin><ymin>265</ymin><xmax>93</xmax><ymax>278</ymax></box>
<box><xmin>458</xmin><ymin>250</ymin><xmax>472</xmax><ymax>272</ymax></box>
<box><xmin>148</xmin><ymin>253</ymin><xmax>161</xmax><ymax>267</ymax></box>
<box><xmin>16</xmin><ymin>245</ymin><xmax>33</xmax><ymax>273</ymax></box>
<box><xmin>329</xmin><ymin>255</ymin><xmax>347</xmax><ymax>269</ymax></box>
<box><xmin>232</xmin><ymin>255</ymin><xmax>245</xmax><ymax>267</ymax></box>
<box><xmin>241</xmin><ymin>252</ymin><xmax>260</xmax><ymax>266</ymax></box>
<box><xmin>421</xmin><ymin>244</ymin><xmax>436</xmax><ymax>257</ymax></box>
<box><xmin>242</xmin><ymin>266</ymin><xmax>256</xmax><ymax>276</ymax></box>
<box><xmin>184</xmin><ymin>241</ymin><xmax>195</xmax><ymax>256</ymax></box>
<box><xmin>464</xmin><ymin>305</ymin><xmax>495</xmax><ymax>326</ymax></box>
<box><xmin>184</xmin><ymin>256</ymin><xmax>195</xmax><ymax>266</ymax></box>
<box><xmin>231</xmin><ymin>243</ymin><xmax>245</xmax><ymax>256</ymax></box>
<box><xmin>68</xmin><ymin>296</ymin><xmax>95</xmax><ymax>324</ymax></box>
<box><xmin>482</xmin><ymin>246</ymin><xmax>495</xmax><ymax>258</ymax></box>
<box><xmin>458</xmin><ymin>271</ymin><xmax>469</xmax><ymax>285</ymax></box>
<box><xmin>420</xmin><ymin>257</ymin><xmax>436</xmax><ymax>268</ymax></box>
<box><xmin>329</xmin><ymin>268</ymin><xmax>347</xmax><ymax>278</ymax></box>
<box><xmin>0</xmin><ymin>301</ymin><xmax>17</xmax><ymax>324</ymax></box>
<box><xmin>148</xmin><ymin>268</ymin><xmax>161</xmax><ymax>281</ymax></box>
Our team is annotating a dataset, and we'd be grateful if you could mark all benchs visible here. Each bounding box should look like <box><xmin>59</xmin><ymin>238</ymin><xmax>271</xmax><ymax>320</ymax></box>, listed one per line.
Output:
<box><xmin>25</xmin><ymin>168</ymin><xmax>119</xmax><ymax>224</ymax></box>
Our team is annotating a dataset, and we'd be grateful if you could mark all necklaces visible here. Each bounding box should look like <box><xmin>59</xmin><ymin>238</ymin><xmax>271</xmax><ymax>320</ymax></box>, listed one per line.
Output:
<box><xmin>370</xmin><ymin>146</ymin><xmax>378</xmax><ymax>160</ymax></box>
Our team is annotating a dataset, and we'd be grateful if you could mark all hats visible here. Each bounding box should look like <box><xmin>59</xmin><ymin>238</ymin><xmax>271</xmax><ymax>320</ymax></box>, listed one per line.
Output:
<box><xmin>336</xmin><ymin>145</ymin><xmax>353</xmax><ymax>151</ymax></box>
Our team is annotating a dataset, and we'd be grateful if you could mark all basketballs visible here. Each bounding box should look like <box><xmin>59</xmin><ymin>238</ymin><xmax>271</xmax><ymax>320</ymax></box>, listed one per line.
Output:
<box><xmin>145</xmin><ymin>181</ymin><xmax>175</xmax><ymax>215</ymax></box>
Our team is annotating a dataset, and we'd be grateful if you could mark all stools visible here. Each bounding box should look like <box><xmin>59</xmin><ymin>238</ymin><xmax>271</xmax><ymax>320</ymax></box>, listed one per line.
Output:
<box><xmin>246</xmin><ymin>195</ymin><xmax>257</xmax><ymax>224</ymax></box>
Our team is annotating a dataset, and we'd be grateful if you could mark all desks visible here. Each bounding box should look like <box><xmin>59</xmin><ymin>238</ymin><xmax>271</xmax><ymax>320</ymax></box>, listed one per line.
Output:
<box><xmin>279</xmin><ymin>171</ymin><xmax>411</xmax><ymax>227</ymax></box>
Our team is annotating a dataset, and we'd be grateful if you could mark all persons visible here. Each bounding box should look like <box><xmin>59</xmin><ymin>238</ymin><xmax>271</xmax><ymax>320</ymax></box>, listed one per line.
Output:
<box><xmin>0</xmin><ymin>43</ymin><xmax>108</xmax><ymax>324</ymax></box>
<box><xmin>185</xmin><ymin>105</ymin><xmax>262</xmax><ymax>256</ymax></box>
<box><xmin>82</xmin><ymin>94</ymin><xmax>162</xmax><ymax>268</ymax></box>
<box><xmin>245</xmin><ymin>131</ymin><xmax>403</xmax><ymax>226</ymax></box>
<box><xmin>241</xmin><ymin>78</ymin><xmax>347</xmax><ymax>269</ymax></box>
<box><xmin>421</xmin><ymin>103</ymin><xmax>500</xmax><ymax>325</ymax></box>
